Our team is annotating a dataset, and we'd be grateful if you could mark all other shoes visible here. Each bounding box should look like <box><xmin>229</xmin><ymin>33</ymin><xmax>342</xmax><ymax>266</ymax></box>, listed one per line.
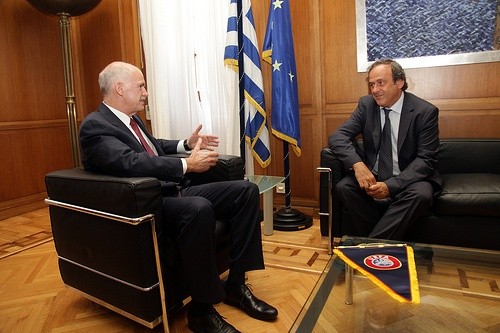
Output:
<box><xmin>334</xmin><ymin>257</ymin><xmax>345</xmax><ymax>269</ymax></box>
<box><xmin>336</xmin><ymin>266</ymin><xmax>356</xmax><ymax>282</ymax></box>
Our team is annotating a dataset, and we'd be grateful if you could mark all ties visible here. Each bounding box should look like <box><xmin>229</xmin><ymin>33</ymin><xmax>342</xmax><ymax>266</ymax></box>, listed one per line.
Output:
<box><xmin>130</xmin><ymin>118</ymin><xmax>181</xmax><ymax>198</ymax></box>
<box><xmin>377</xmin><ymin>105</ymin><xmax>393</xmax><ymax>182</ymax></box>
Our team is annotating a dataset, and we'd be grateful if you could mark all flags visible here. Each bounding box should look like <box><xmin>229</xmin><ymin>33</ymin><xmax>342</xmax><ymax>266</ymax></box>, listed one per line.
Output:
<box><xmin>261</xmin><ymin>0</ymin><xmax>301</xmax><ymax>158</ymax></box>
<box><xmin>223</xmin><ymin>0</ymin><xmax>271</xmax><ymax>168</ymax></box>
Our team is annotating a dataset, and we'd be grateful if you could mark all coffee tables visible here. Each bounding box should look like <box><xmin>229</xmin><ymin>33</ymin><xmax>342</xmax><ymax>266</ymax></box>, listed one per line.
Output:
<box><xmin>246</xmin><ymin>175</ymin><xmax>286</xmax><ymax>236</ymax></box>
<box><xmin>287</xmin><ymin>235</ymin><xmax>500</xmax><ymax>333</ymax></box>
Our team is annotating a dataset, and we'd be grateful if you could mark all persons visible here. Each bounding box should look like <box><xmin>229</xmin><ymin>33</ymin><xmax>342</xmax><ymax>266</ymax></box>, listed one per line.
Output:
<box><xmin>327</xmin><ymin>59</ymin><xmax>440</xmax><ymax>240</ymax></box>
<box><xmin>79</xmin><ymin>61</ymin><xmax>277</xmax><ymax>333</ymax></box>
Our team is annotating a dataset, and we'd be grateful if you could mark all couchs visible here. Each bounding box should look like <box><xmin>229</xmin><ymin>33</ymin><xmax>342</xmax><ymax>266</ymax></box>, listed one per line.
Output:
<box><xmin>44</xmin><ymin>152</ymin><xmax>246</xmax><ymax>333</ymax></box>
<box><xmin>317</xmin><ymin>137</ymin><xmax>500</xmax><ymax>253</ymax></box>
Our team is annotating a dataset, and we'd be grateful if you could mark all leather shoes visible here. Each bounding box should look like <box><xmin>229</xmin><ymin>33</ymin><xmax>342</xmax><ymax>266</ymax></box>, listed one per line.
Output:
<box><xmin>222</xmin><ymin>282</ymin><xmax>278</xmax><ymax>320</ymax></box>
<box><xmin>189</xmin><ymin>305</ymin><xmax>242</xmax><ymax>333</ymax></box>
